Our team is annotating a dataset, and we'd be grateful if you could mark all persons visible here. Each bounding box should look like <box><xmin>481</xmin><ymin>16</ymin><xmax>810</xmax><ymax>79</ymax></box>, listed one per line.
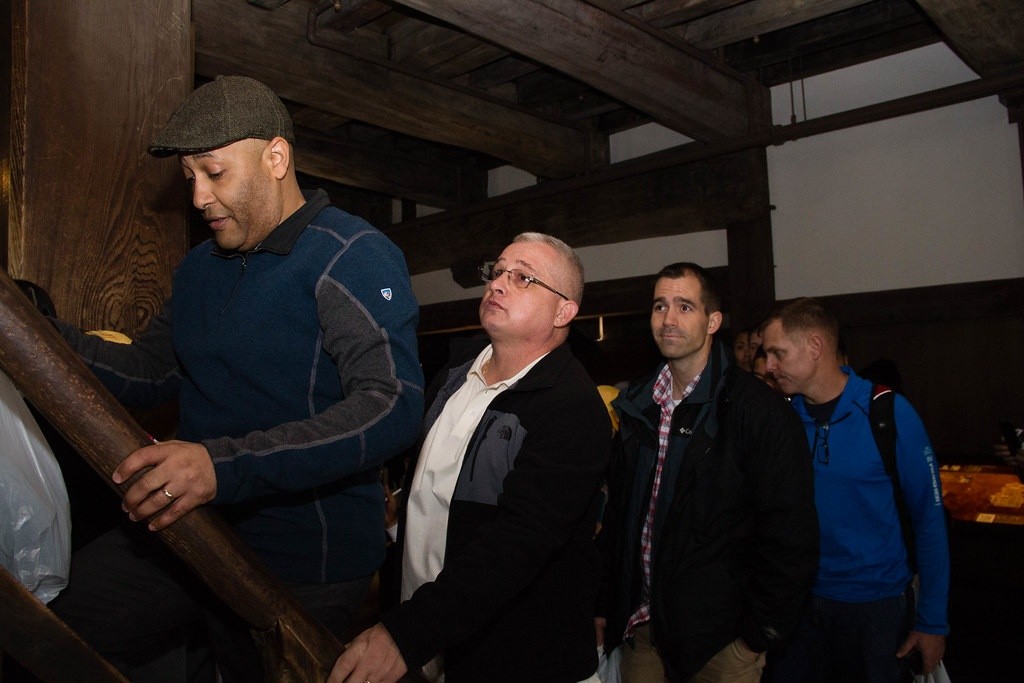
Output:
<box><xmin>597</xmin><ymin>263</ymin><xmax>819</xmax><ymax>683</ymax></box>
<box><xmin>736</xmin><ymin>298</ymin><xmax>947</xmax><ymax>683</ymax></box>
<box><xmin>42</xmin><ymin>74</ymin><xmax>422</xmax><ymax>683</ymax></box>
<box><xmin>319</xmin><ymin>232</ymin><xmax>614</xmax><ymax>683</ymax></box>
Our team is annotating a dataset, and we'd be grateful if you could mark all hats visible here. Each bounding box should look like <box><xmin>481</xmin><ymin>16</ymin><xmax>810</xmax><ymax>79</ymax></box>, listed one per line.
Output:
<box><xmin>146</xmin><ymin>74</ymin><xmax>295</xmax><ymax>158</ymax></box>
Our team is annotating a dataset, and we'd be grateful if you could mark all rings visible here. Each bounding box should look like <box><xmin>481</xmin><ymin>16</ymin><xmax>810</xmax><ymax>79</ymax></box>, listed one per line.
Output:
<box><xmin>161</xmin><ymin>488</ymin><xmax>175</xmax><ymax>501</ymax></box>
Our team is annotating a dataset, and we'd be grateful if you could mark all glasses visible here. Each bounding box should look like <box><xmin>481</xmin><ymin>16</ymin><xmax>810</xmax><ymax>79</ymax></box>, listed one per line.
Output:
<box><xmin>477</xmin><ymin>261</ymin><xmax>569</xmax><ymax>303</ymax></box>
<box><xmin>753</xmin><ymin>372</ymin><xmax>777</xmax><ymax>386</ymax></box>
<box><xmin>816</xmin><ymin>422</ymin><xmax>830</xmax><ymax>464</ymax></box>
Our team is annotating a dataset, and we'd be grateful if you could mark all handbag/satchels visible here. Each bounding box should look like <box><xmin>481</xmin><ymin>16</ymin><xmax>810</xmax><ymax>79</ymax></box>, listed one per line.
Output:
<box><xmin>0</xmin><ymin>370</ymin><xmax>71</xmax><ymax>606</ymax></box>
